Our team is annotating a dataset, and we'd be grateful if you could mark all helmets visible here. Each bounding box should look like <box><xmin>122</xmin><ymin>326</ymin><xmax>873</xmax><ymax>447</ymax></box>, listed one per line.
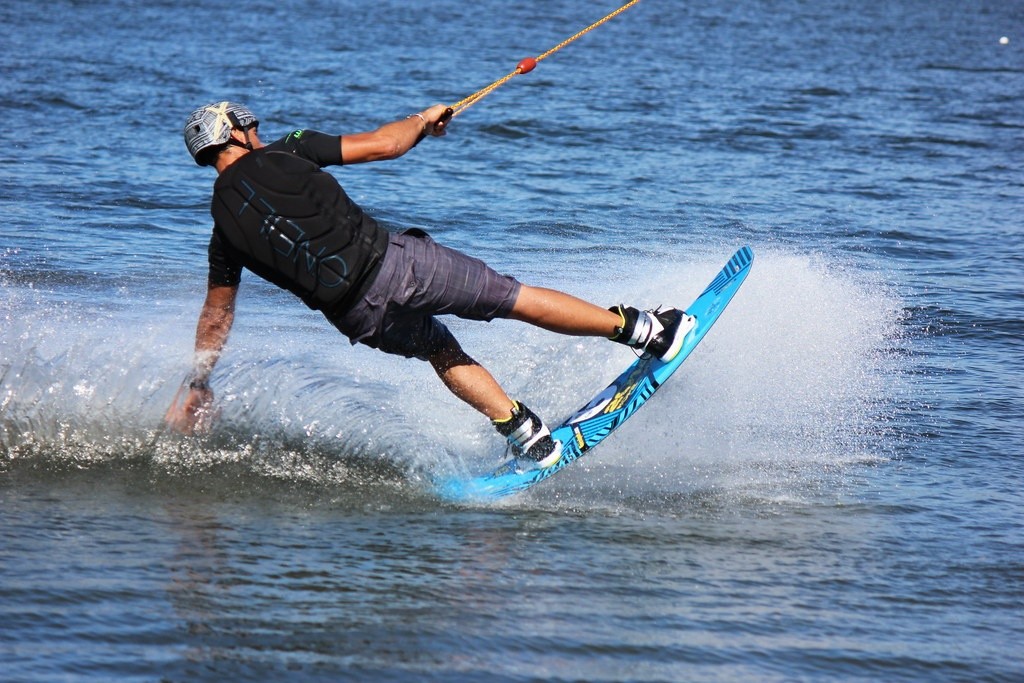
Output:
<box><xmin>184</xmin><ymin>99</ymin><xmax>260</xmax><ymax>167</ymax></box>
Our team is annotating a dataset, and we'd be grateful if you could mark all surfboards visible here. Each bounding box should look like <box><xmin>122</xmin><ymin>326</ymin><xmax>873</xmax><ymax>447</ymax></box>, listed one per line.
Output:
<box><xmin>485</xmin><ymin>245</ymin><xmax>754</xmax><ymax>497</ymax></box>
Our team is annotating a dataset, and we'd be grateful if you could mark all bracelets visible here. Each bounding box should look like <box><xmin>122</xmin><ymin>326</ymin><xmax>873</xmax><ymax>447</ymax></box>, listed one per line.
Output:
<box><xmin>407</xmin><ymin>113</ymin><xmax>427</xmax><ymax>132</ymax></box>
<box><xmin>186</xmin><ymin>378</ymin><xmax>210</xmax><ymax>391</ymax></box>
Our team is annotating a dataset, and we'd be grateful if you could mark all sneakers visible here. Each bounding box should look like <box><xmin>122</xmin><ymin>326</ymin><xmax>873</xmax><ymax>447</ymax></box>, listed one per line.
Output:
<box><xmin>490</xmin><ymin>400</ymin><xmax>562</xmax><ymax>471</ymax></box>
<box><xmin>607</xmin><ymin>302</ymin><xmax>691</xmax><ymax>364</ymax></box>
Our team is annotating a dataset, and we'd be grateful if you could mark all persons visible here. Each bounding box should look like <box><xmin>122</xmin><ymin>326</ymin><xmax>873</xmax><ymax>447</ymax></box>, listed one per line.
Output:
<box><xmin>173</xmin><ymin>100</ymin><xmax>697</xmax><ymax>470</ymax></box>
